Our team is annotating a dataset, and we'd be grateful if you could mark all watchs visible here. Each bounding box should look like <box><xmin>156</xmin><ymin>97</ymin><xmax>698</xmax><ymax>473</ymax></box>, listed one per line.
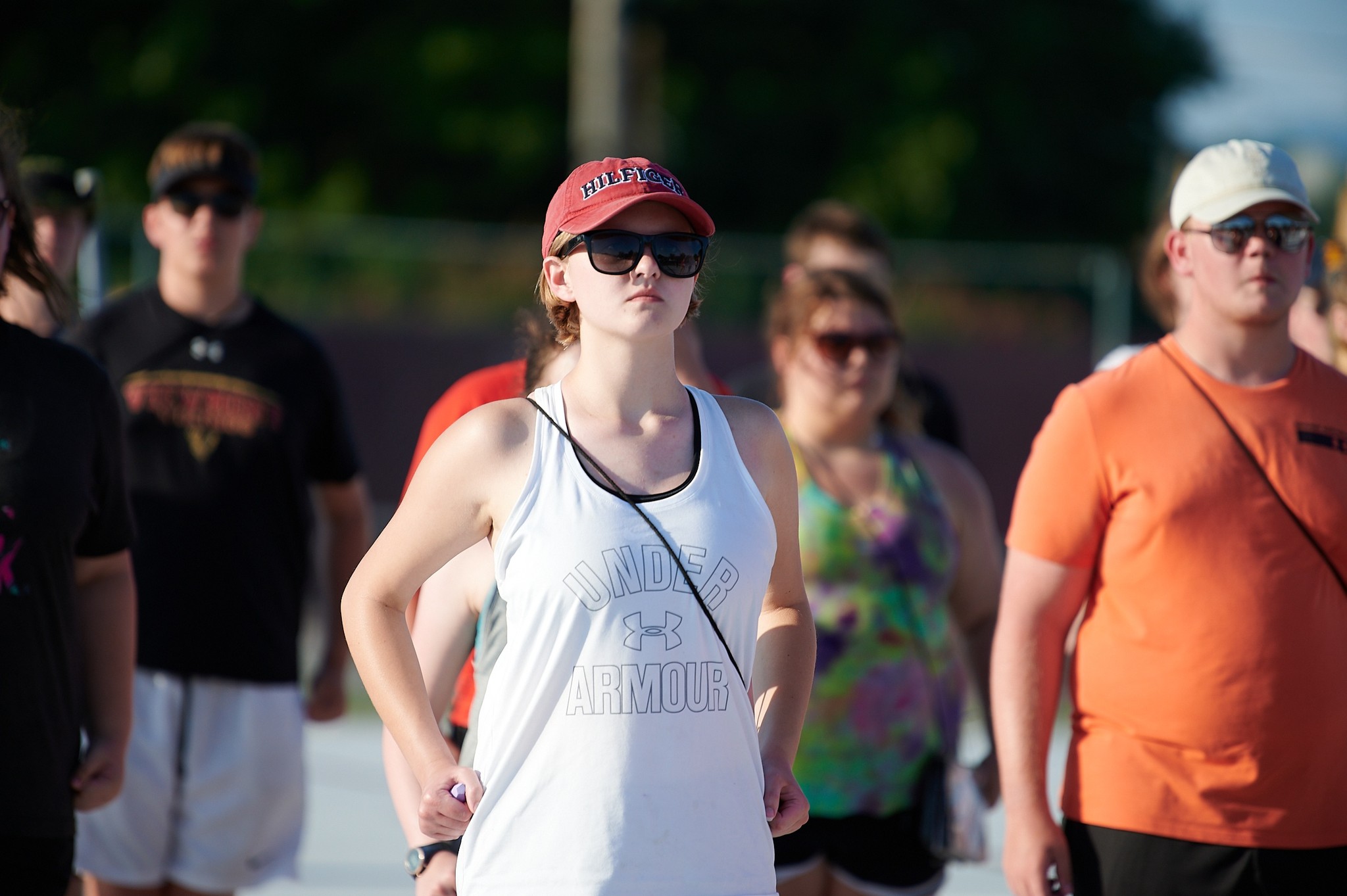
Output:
<box><xmin>404</xmin><ymin>841</ymin><xmax>458</xmax><ymax>881</ymax></box>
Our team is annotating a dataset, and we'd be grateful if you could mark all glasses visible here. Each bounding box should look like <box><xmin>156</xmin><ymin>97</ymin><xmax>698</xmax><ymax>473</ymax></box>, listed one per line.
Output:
<box><xmin>1181</xmin><ymin>213</ymin><xmax>1318</xmax><ymax>256</ymax></box>
<box><xmin>559</xmin><ymin>227</ymin><xmax>709</xmax><ymax>277</ymax></box>
<box><xmin>809</xmin><ymin>328</ymin><xmax>893</xmax><ymax>362</ymax></box>
<box><xmin>166</xmin><ymin>181</ymin><xmax>249</xmax><ymax>220</ymax></box>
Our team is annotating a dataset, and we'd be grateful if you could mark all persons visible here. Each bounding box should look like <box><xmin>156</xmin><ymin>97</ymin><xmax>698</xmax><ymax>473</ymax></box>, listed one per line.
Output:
<box><xmin>341</xmin><ymin>157</ymin><xmax>818</xmax><ymax>896</ymax></box>
<box><xmin>989</xmin><ymin>139</ymin><xmax>1347</xmax><ymax>896</ymax></box>
<box><xmin>385</xmin><ymin>204</ymin><xmax>1006</xmax><ymax>896</ymax></box>
<box><xmin>0</xmin><ymin>125</ymin><xmax>368</xmax><ymax>896</ymax></box>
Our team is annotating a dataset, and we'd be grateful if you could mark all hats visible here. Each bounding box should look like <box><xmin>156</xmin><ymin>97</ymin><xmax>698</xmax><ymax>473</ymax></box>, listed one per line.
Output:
<box><xmin>541</xmin><ymin>156</ymin><xmax>717</xmax><ymax>262</ymax></box>
<box><xmin>147</xmin><ymin>163</ymin><xmax>259</xmax><ymax>202</ymax></box>
<box><xmin>1168</xmin><ymin>138</ymin><xmax>1323</xmax><ymax>230</ymax></box>
